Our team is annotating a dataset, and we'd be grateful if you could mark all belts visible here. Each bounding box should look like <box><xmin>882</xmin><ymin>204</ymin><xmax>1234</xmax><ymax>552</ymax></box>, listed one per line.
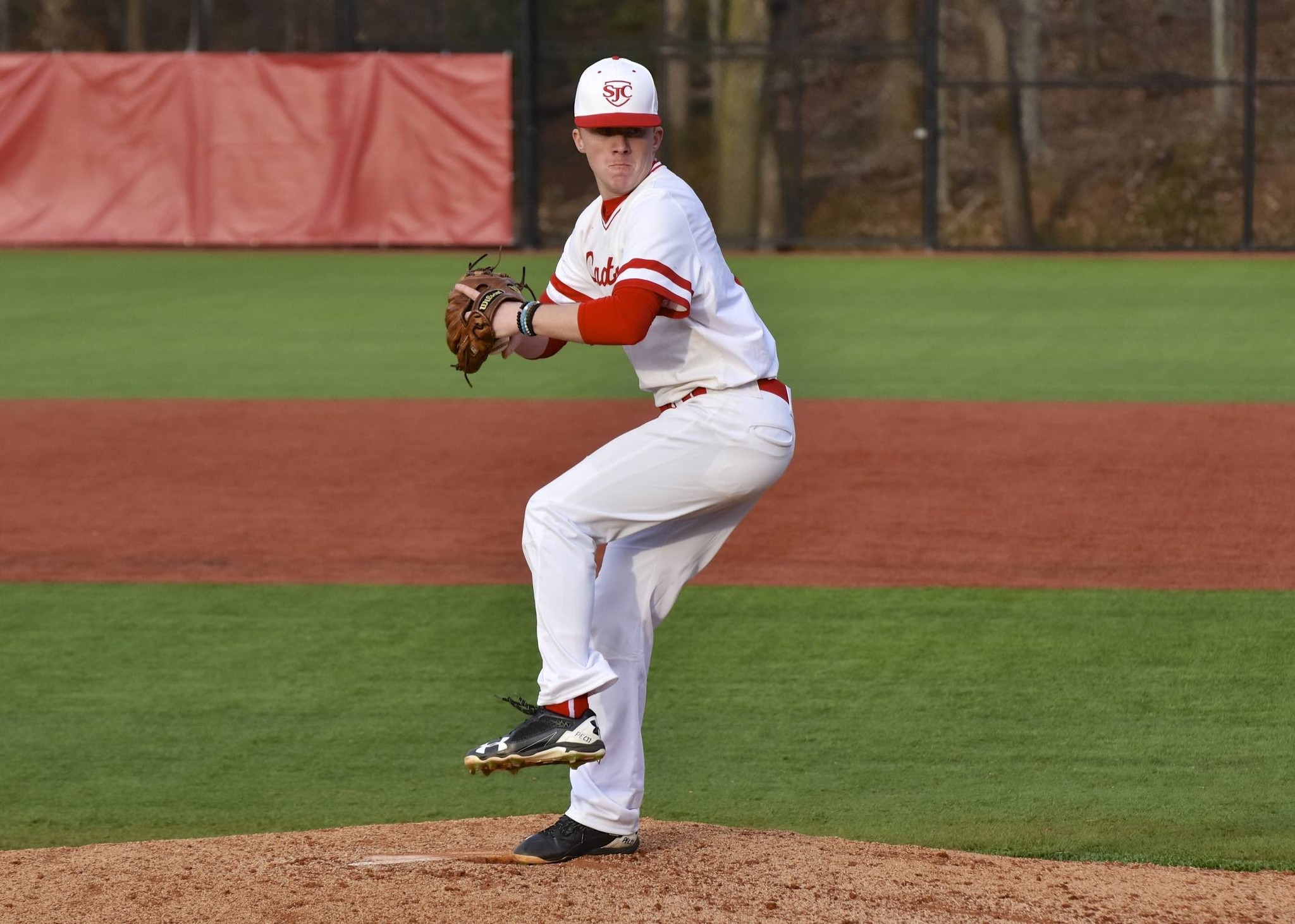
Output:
<box><xmin>659</xmin><ymin>375</ymin><xmax>788</xmax><ymax>411</ymax></box>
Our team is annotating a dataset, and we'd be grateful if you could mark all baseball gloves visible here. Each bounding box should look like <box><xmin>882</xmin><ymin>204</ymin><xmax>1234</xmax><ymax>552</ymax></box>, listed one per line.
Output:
<box><xmin>445</xmin><ymin>268</ymin><xmax>527</xmax><ymax>374</ymax></box>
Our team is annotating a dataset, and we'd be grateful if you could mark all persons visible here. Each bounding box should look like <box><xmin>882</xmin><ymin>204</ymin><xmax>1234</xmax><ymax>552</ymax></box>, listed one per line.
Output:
<box><xmin>445</xmin><ymin>57</ymin><xmax>796</xmax><ymax>865</ymax></box>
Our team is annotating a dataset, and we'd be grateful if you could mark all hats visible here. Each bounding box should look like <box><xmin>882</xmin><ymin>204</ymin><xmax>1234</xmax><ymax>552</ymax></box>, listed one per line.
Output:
<box><xmin>573</xmin><ymin>56</ymin><xmax>661</xmax><ymax>128</ymax></box>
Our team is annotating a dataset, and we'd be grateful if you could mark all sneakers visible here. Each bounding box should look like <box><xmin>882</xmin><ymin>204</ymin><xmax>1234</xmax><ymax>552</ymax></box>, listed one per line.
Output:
<box><xmin>512</xmin><ymin>813</ymin><xmax>640</xmax><ymax>864</ymax></box>
<box><xmin>464</xmin><ymin>696</ymin><xmax>605</xmax><ymax>775</ymax></box>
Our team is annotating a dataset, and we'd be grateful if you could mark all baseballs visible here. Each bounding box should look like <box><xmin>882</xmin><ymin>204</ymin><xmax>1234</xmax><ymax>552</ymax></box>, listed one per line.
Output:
<box><xmin>488</xmin><ymin>337</ymin><xmax>510</xmax><ymax>355</ymax></box>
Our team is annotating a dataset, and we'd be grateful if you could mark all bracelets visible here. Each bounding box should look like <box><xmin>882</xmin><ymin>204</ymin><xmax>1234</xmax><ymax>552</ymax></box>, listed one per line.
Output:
<box><xmin>516</xmin><ymin>301</ymin><xmax>541</xmax><ymax>336</ymax></box>
